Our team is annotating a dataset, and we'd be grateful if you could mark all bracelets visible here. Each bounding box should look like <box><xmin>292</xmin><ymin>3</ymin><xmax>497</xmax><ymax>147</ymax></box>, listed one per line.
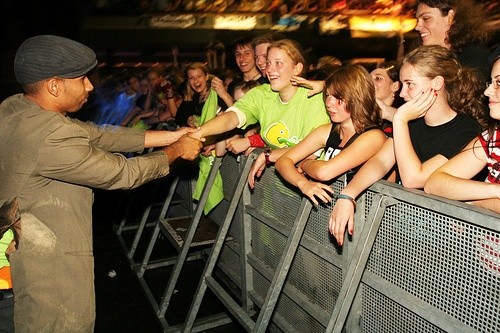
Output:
<box><xmin>334</xmin><ymin>194</ymin><xmax>357</xmax><ymax>209</ymax></box>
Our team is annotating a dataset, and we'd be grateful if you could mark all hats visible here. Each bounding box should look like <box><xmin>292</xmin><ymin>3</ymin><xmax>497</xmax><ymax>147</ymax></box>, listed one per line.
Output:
<box><xmin>14</xmin><ymin>35</ymin><xmax>98</xmax><ymax>84</ymax></box>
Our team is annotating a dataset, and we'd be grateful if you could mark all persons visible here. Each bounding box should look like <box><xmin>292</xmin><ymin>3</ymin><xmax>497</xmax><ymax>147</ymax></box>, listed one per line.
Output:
<box><xmin>83</xmin><ymin>1</ymin><xmax>500</xmax><ymax>277</ymax></box>
<box><xmin>0</xmin><ymin>35</ymin><xmax>203</xmax><ymax>332</ymax></box>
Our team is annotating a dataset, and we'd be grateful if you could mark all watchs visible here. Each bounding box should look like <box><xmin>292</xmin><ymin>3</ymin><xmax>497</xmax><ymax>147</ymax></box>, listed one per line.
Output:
<box><xmin>264</xmin><ymin>147</ymin><xmax>272</xmax><ymax>165</ymax></box>
